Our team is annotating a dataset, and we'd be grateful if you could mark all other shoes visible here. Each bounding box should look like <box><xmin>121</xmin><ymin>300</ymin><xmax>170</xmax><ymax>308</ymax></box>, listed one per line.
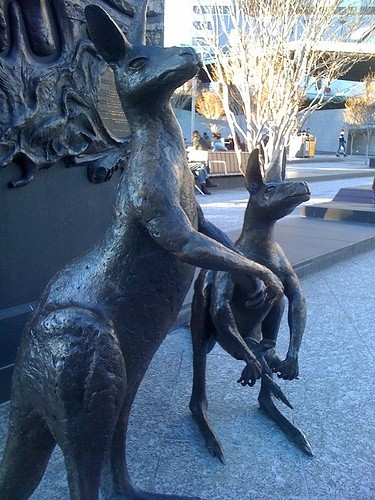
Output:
<box><xmin>206</xmin><ymin>182</ymin><xmax>218</xmax><ymax>187</ymax></box>
<box><xmin>200</xmin><ymin>189</ymin><xmax>211</xmax><ymax>195</ymax></box>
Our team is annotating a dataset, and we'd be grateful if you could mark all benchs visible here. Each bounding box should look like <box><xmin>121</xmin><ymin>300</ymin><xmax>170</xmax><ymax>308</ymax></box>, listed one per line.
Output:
<box><xmin>207</xmin><ymin>152</ymin><xmax>249</xmax><ymax>178</ymax></box>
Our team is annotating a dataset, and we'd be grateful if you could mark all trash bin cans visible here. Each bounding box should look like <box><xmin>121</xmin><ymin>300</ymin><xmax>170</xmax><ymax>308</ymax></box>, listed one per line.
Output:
<box><xmin>288</xmin><ymin>132</ymin><xmax>315</xmax><ymax>158</ymax></box>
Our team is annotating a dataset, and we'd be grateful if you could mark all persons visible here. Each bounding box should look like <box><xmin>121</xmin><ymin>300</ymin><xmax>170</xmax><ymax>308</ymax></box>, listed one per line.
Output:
<box><xmin>193</xmin><ymin>126</ymin><xmax>312</xmax><ymax>153</ymax></box>
<box><xmin>336</xmin><ymin>129</ymin><xmax>348</xmax><ymax>157</ymax></box>
<box><xmin>187</xmin><ymin>162</ymin><xmax>220</xmax><ymax>195</ymax></box>
